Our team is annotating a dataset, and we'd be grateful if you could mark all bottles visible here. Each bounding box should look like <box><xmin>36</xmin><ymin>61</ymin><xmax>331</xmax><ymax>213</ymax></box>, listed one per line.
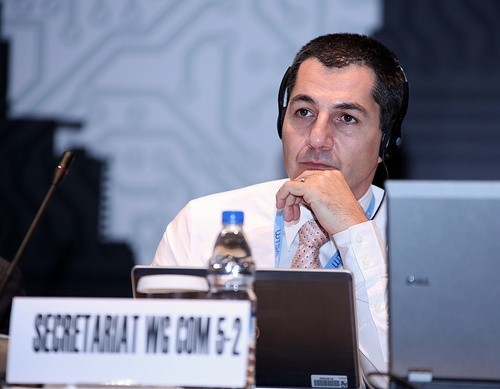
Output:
<box><xmin>206</xmin><ymin>211</ymin><xmax>257</xmax><ymax>389</ymax></box>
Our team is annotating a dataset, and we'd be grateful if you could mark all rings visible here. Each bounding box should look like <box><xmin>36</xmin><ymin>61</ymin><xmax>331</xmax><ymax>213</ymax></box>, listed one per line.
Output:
<box><xmin>301</xmin><ymin>177</ymin><xmax>307</xmax><ymax>182</ymax></box>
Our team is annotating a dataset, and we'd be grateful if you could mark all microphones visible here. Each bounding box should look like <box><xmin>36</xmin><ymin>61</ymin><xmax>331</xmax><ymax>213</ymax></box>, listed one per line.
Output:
<box><xmin>0</xmin><ymin>152</ymin><xmax>74</xmax><ymax>300</ymax></box>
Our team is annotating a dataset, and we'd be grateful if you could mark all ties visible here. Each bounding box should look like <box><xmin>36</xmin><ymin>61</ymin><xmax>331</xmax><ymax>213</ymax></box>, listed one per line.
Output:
<box><xmin>289</xmin><ymin>220</ymin><xmax>328</xmax><ymax>269</ymax></box>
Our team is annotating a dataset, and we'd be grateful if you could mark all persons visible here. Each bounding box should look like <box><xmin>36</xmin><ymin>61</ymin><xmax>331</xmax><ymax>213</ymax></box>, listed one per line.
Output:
<box><xmin>149</xmin><ymin>32</ymin><xmax>408</xmax><ymax>389</ymax></box>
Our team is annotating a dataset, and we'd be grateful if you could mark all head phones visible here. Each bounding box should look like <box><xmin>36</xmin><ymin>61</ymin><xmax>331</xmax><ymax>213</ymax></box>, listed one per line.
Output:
<box><xmin>277</xmin><ymin>42</ymin><xmax>409</xmax><ymax>157</ymax></box>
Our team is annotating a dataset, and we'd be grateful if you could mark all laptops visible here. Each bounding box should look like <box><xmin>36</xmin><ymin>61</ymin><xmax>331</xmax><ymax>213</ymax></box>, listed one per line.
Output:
<box><xmin>133</xmin><ymin>266</ymin><xmax>361</xmax><ymax>389</ymax></box>
<box><xmin>384</xmin><ymin>180</ymin><xmax>500</xmax><ymax>389</ymax></box>
